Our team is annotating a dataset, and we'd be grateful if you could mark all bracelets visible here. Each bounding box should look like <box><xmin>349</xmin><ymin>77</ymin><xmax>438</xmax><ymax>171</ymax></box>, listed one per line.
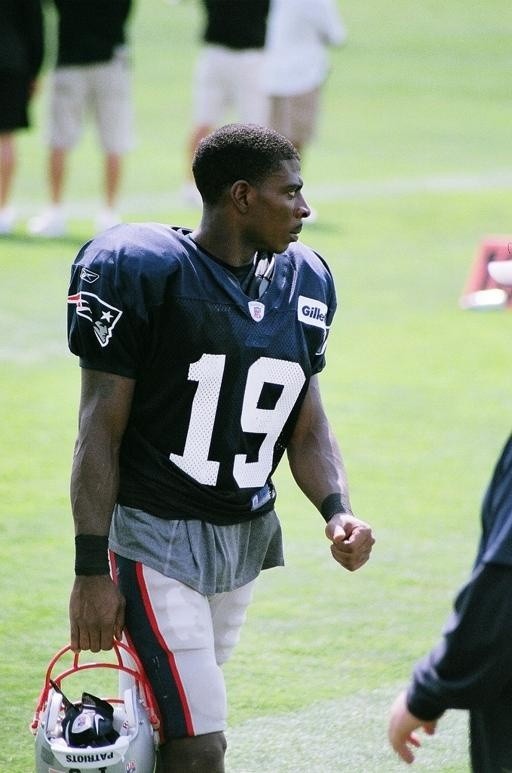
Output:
<box><xmin>320</xmin><ymin>493</ymin><xmax>355</xmax><ymax>523</ymax></box>
<box><xmin>74</xmin><ymin>534</ymin><xmax>110</xmax><ymax>576</ymax></box>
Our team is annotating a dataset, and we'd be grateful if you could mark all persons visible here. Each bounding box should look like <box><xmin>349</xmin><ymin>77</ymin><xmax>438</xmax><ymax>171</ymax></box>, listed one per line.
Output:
<box><xmin>186</xmin><ymin>1</ymin><xmax>346</xmax><ymax>160</ymax></box>
<box><xmin>387</xmin><ymin>434</ymin><xmax>512</xmax><ymax>773</ymax></box>
<box><xmin>0</xmin><ymin>0</ymin><xmax>135</xmax><ymax>235</ymax></box>
<box><xmin>69</xmin><ymin>123</ymin><xmax>377</xmax><ymax>773</ymax></box>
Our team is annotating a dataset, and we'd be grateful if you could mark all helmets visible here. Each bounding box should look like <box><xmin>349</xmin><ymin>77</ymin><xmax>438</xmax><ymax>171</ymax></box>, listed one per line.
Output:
<box><xmin>29</xmin><ymin>640</ymin><xmax>166</xmax><ymax>773</ymax></box>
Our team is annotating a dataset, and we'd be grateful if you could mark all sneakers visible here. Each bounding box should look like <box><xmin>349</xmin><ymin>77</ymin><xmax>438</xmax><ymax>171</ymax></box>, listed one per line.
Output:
<box><xmin>2</xmin><ymin>199</ymin><xmax>127</xmax><ymax>241</ymax></box>
